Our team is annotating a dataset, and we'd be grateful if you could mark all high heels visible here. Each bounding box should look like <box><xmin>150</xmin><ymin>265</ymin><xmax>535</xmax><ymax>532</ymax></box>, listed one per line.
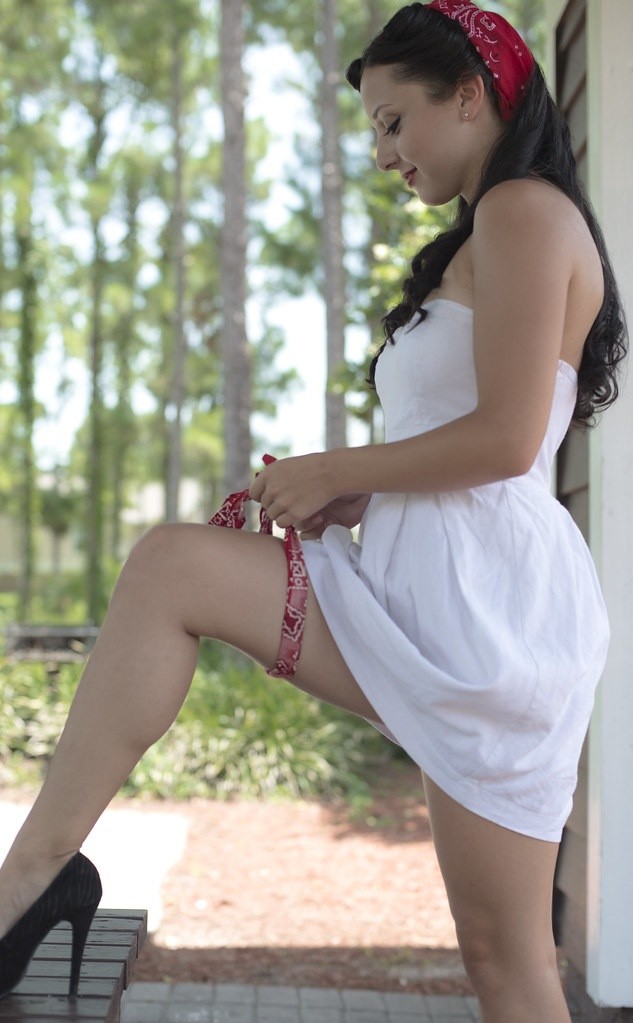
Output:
<box><xmin>0</xmin><ymin>851</ymin><xmax>103</xmax><ymax>1001</ymax></box>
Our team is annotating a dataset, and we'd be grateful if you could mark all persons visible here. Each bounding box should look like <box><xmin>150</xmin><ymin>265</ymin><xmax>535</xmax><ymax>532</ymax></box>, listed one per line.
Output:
<box><xmin>0</xmin><ymin>0</ymin><xmax>631</xmax><ymax>1023</ymax></box>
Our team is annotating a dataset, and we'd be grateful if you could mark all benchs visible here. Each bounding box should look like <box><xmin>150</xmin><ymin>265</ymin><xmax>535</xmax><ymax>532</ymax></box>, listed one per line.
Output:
<box><xmin>0</xmin><ymin>910</ymin><xmax>147</xmax><ymax>1022</ymax></box>
